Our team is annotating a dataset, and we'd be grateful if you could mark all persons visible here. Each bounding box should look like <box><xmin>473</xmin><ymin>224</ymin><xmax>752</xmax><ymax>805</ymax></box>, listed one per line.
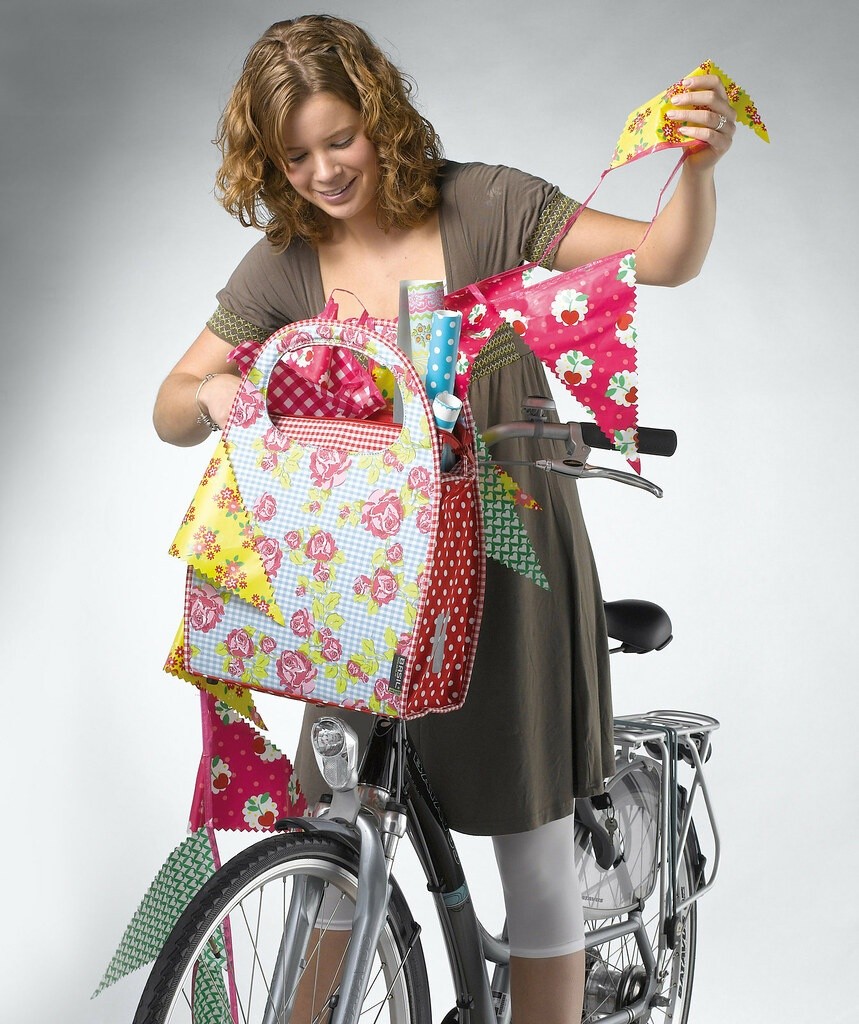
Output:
<box><xmin>153</xmin><ymin>12</ymin><xmax>738</xmax><ymax>1024</ymax></box>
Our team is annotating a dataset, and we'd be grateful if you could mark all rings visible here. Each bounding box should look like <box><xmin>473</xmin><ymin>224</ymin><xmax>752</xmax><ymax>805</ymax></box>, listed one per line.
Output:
<box><xmin>715</xmin><ymin>114</ymin><xmax>726</xmax><ymax>130</ymax></box>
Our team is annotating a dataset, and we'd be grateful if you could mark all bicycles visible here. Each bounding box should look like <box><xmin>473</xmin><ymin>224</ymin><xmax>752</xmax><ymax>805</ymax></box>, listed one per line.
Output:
<box><xmin>134</xmin><ymin>393</ymin><xmax>720</xmax><ymax>1024</ymax></box>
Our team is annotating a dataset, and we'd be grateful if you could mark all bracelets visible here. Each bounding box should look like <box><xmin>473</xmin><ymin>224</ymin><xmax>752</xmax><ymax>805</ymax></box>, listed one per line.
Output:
<box><xmin>195</xmin><ymin>374</ymin><xmax>221</xmax><ymax>431</ymax></box>
